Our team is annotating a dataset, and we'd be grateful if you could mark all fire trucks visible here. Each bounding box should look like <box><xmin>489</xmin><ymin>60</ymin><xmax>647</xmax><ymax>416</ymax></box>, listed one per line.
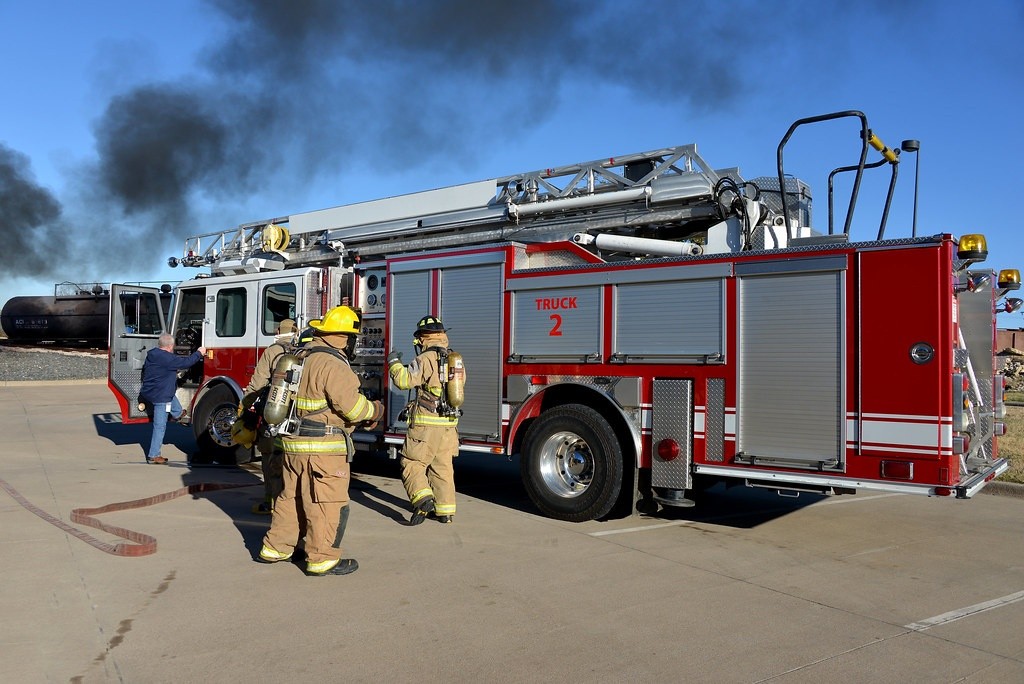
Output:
<box><xmin>101</xmin><ymin>105</ymin><xmax>1022</xmax><ymax>521</ymax></box>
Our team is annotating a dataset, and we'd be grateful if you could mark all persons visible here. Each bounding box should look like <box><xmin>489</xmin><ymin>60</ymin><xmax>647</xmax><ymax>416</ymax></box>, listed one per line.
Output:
<box><xmin>138</xmin><ymin>333</ymin><xmax>206</xmax><ymax>464</ymax></box>
<box><xmin>237</xmin><ymin>305</ymin><xmax>384</xmax><ymax>578</ymax></box>
<box><xmin>386</xmin><ymin>316</ymin><xmax>466</xmax><ymax>526</ymax></box>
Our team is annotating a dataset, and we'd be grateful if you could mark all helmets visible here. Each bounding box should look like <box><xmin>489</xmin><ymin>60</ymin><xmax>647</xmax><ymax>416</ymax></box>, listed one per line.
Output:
<box><xmin>232</xmin><ymin>420</ymin><xmax>257</xmax><ymax>450</ymax></box>
<box><xmin>308</xmin><ymin>305</ymin><xmax>360</xmax><ymax>334</ymax></box>
<box><xmin>413</xmin><ymin>316</ymin><xmax>444</xmax><ymax>337</ymax></box>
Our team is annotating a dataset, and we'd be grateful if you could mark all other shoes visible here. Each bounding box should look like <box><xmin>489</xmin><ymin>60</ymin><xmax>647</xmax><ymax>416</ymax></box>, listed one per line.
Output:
<box><xmin>174</xmin><ymin>409</ymin><xmax>187</xmax><ymax>421</ymax></box>
<box><xmin>147</xmin><ymin>457</ymin><xmax>168</xmax><ymax>464</ymax></box>
<box><xmin>251</xmin><ymin>502</ymin><xmax>274</xmax><ymax>514</ymax></box>
<box><xmin>426</xmin><ymin>511</ymin><xmax>452</xmax><ymax>523</ymax></box>
<box><xmin>257</xmin><ymin>548</ymin><xmax>308</xmax><ymax>563</ymax></box>
<box><xmin>410</xmin><ymin>499</ymin><xmax>434</xmax><ymax>525</ymax></box>
<box><xmin>306</xmin><ymin>559</ymin><xmax>358</xmax><ymax>576</ymax></box>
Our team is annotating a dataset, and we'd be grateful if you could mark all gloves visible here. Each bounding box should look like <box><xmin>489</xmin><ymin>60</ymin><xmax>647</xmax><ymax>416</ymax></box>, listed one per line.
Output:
<box><xmin>362</xmin><ymin>421</ymin><xmax>378</xmax><ymax>431</ymax></box>
<box><xmin>237</xmin><ymin>401</ymin><xmax>246</xmax><ymax>417</ymax></box>
<box><xmin>371</xmin><ymin>400</ymin><xmax>384</xmax><ymax>423</ymax></box>
<box><xmin>387</xmin><ymin>351</ymin><xmax>402</xmax><ymax>369</ymax></box>
